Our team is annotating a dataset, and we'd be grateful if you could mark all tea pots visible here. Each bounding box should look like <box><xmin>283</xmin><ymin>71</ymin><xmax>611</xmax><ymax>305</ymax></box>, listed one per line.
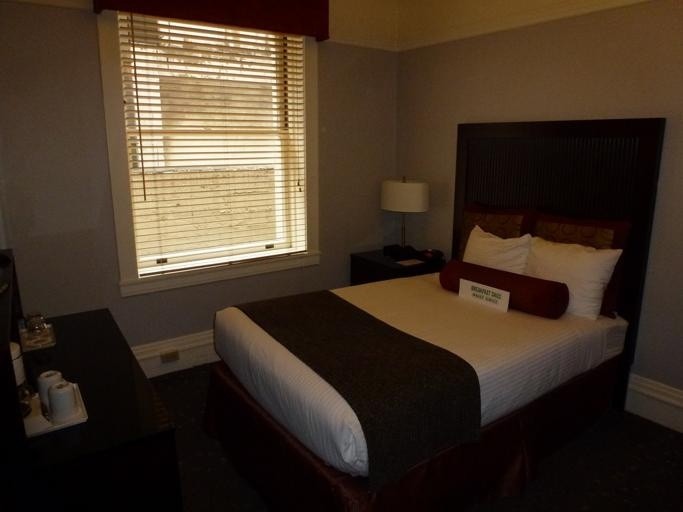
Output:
<box><xmin>25</xmin><ymin>311</ymin><xmax>50</xmax><ymax>345</ymax></box>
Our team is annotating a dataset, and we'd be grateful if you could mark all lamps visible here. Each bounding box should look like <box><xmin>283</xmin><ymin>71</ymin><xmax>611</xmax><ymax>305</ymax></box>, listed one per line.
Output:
<box><xmin>381</xmin><ymin>178</ymin><xmax>429</xmax><ymax>261</ymax></box>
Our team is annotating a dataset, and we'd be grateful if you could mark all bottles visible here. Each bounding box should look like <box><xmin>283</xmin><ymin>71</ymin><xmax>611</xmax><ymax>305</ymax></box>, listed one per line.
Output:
<box><xmin>38</xmin><ymin>370</ymin><xmax>82</xmax><ymax>426</ymax></box>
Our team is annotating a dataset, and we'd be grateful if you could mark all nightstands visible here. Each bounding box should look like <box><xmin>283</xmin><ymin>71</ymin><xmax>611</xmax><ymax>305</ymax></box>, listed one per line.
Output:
<box><xmin>348</xmin><ymin>248</ymin><xmax>446</xmax><ymax>285</ymax></box>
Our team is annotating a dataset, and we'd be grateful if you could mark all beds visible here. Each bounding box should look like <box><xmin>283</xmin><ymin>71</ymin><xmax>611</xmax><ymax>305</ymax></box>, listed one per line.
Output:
<box><xmin>209</xmin><ymin>116</ymin><xmax>667</xmax><ymax>511</ymax></box>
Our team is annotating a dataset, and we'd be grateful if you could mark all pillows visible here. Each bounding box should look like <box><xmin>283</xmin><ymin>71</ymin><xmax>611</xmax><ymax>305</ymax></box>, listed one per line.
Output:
<box><xmin>438</xmin><ymin>203</ymin><xmax>629</xmax><ymax>324</ymax></box>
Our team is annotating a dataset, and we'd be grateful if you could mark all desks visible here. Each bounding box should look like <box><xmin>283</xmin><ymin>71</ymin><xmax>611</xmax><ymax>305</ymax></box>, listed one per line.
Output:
<box><xmin>3</xmin><ymin>306</ymin><xmax>185</xmax><ymax>512</ymax></box>
